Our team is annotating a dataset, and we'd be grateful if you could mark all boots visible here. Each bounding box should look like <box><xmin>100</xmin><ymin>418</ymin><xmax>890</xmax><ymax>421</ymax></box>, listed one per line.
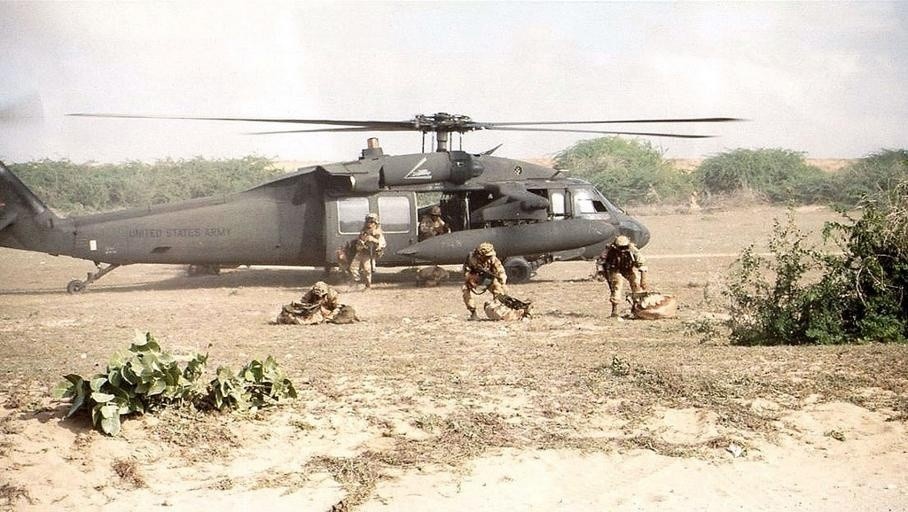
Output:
<box><xmin>351</xmin><ymin>281</ymin><xmax>372</xmax><ymax>289</ymax></box>
<box><xmin>468</xmin><ymin>310</ymin><xmax>479</xmax><ymax>321</ymax></box>
<box><xmin>611</xmin><ymin>303</ymin><xmax>619</xmax><ymax>317</ymax></box>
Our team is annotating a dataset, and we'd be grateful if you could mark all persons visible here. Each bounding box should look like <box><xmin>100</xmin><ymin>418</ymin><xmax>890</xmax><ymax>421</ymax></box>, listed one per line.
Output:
<box><xmin>596</xmin><ymin>236</ymin><xmax>648</xmax><ymax>316</ymax></box>
<box><xmin>463</xmin><ymin>243</ymin><xmax>508</xmax><ymax>320</ymax></box>
<box><xmin>420</xmin><ymin>206</ymin><xmax>451</xmax><ymax>238</ymax></box>
<box><xmin>277</xmin><ymin>282</ymin><xmax>342</xmax><ymax>324</ymax></box>
<box><xmin>346</xmin><ymin>212</ymin><xmax>387</xmax><ymax>294</ymax></box>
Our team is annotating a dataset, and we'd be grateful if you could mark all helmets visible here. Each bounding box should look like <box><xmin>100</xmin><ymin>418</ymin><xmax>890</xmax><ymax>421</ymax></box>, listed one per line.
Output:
<box><xmin>312</xmin><ymin>281</ymin><xmax>328</xmax><ymax>295</ymax></box>
<box><xmin>479</xmin><ymin>242</ymin><xmax>497</xmax><ymax>257</ymax></box>
<box><xmin>429</xmin><ymin>206</ymin><xmax>441</xmax><ymax>216</ymax></box>
<box><xmin>613</xmin><ymin>235</ymin><xmax>630</xmax><ymax>250</ymax></box>
<box><xmin>365</xmin><ymin>212</ymin><xmax>378</xmax><ymax>222</ymax></box>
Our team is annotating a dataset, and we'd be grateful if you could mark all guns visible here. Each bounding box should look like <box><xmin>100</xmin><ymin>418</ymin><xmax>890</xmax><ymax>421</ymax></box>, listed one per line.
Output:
<box><xmin>367</xmin><ymin>241</ymin><xmax>373</xmax><ymax>273</ymax></box>
<box><xmin>470</xmin><ymin>267</ymin><xmax>502</xmax><ymax>280</ymax></box>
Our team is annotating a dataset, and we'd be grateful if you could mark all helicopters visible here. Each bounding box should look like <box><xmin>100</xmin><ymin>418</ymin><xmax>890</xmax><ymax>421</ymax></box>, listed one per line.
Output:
<box><xmin>0</xmin><ymin>113</ymin><xmax>745</xmax><ymax>294</ymax></box>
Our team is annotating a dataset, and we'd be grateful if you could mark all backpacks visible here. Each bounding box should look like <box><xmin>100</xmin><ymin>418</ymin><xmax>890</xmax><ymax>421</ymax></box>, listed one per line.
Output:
<box><xmin>484</xmin><ymin>294</ymin><xmax>534</xmax><ymax>321</ymax></box>
<box><xmin>335</xmin><ymin>238</ymin><xmax>359</xmax><ymax>272</ymax></box>
<box><xmin>276</xmin><ymin>302</ymin><xmax>322</xmax><ymax>324</ymax></box>
<box><xmin>626</xmin><ymin>290</ymin><xmax>678</xmax><ymax>319</ymax></box>
